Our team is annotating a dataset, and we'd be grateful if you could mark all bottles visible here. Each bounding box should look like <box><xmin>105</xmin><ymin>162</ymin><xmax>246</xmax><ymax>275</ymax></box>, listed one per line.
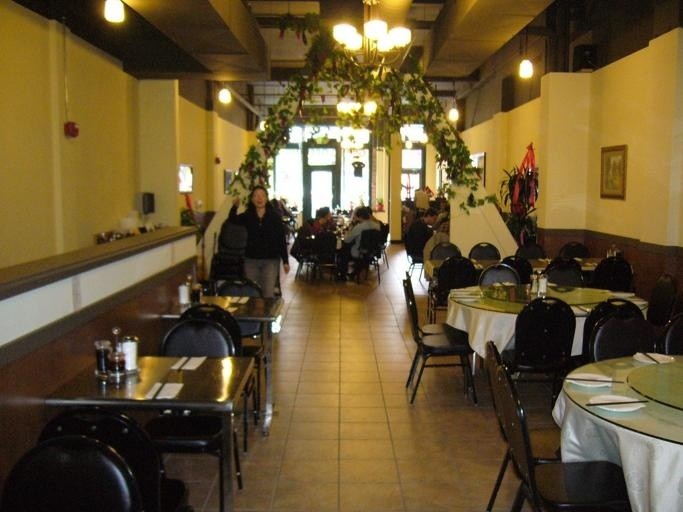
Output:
<box><xmin>177</xmin><ymin>274</ymin><xmax>201</xmax><ymax>305</ymax></box>
<box><xmin>491</xmin><ymin>272</ymin><xmax>548</xmax><ymax>301</ymax></box>
<box><xmin>92</xmin><ymin>328</ymin><xmax>138</xmax><ymax>381</ymax></box>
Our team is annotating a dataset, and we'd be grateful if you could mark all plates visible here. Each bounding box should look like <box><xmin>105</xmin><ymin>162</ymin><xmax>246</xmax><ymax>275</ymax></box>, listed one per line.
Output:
<box><xmin>567</xmin><ymin>374</ymin><xmax>609</xmax><ymax>388</ymax></box>
<box><xmin>631</xmin><ymin>352</ymin><xmax>673</xmax><ymax>365</ymax></box>
<box><xmin>589</xmin><ymin>395</ymin><xmax>643</xmax><ymax>413</ymax></box>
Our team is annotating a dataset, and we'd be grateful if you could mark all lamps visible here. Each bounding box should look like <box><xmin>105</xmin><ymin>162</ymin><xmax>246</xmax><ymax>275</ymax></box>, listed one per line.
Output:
<box><xmin>336</xmin><ymin>85</ymin><xmax>394</xmax><ymax>117</ymax></box>
<box><xmin>259</xmin><ymin>80</ymin><xmax>267</xmax><ymax>131</ymax></box>
<box><xmin>218</xmin><ymin>81</ymin><xmax>232</xmax><ymax>105</ymax></box>
<box><xmin>104</xmin><ymin>0</ymin><xmax>126</xmax><ymax>24</ymax></box>
<box><xmin>446</xmin><ymin>81</ymin><xmax>460</xmax><ymax>123</ymax></box>
<box><xmin>332</xmin><ymin>0</ymin><xmax>412</xmax><ymax>68</ymax></box>
<box><xmin>518</xmin><ymin>25</ymin><xmax>535</xmax><ymax>80</ymax></box>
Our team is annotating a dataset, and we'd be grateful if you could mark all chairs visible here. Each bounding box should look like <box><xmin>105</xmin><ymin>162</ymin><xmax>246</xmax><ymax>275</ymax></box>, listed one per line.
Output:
<box><xmin>485</xmin><ymin>340</ymin><xmax>631</xmax><ymax>511</ymax></box>
<box><xmin>179</xmin><ymin>303</ymin><xmax>266</xmax><ymax>381</ymax></box>
<box><xmin>215</xmin><ymin>275</ymin><xmax>268</xmax><ymax>337</ymax></box>
<box><xmin>1</xmin><ymin>402</ymin><xmax>167</xmax><ymax>511</ymax></box>
<box><xmin>149</xmin><ymin>312</ymin><xmax>243</xmax><ymax>511</ymax></box>
<box><xmin>294</xmin><ymin>222</ymin><xmax>390</xmax><ymax>284</ymax></box>
<box><xmin>403</xmin><ymin>241</ymin><xmax>683</xmax><ymax>410</ymax></box>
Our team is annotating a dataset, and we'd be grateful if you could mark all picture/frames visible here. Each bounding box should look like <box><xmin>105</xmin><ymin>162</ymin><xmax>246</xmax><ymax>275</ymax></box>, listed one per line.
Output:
<box><xmin>599</xmin><ymin>144</ymin><xmax>628</xmax><ymax>200</ymax></box>
<box><xmin>224</xmin><ymin>169</ymin><xmax>233</xmax><ymax>195</ymax></box>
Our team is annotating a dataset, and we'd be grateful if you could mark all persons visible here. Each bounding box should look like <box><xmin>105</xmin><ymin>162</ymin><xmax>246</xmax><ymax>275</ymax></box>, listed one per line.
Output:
<box><xmin>229</xmin><ymin>186</ymin><xmax>289</xmax><ymax>297</ymax></box>
<box><xmin>270</xmin><ymin>197</ymin><xmax>450</xmax><ymax>281</ymax></box>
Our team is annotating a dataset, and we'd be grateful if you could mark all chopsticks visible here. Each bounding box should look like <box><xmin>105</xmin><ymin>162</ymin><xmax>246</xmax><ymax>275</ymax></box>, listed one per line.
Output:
<box><xmin>150</xmin><ymin>379</ymin><xmax>167</xmax><ymax>400</ymax></box>
<box><xmin>560</xmin><ymin>378</ymin><xmax>624</xmax><ymax>383</ymax></box>
<box><xmin>638</xmin><ymin>350</ymin><xmax>660</xmax><ymax>365</ymax></box>
<box><xmin>176</xmin><ymin>354</ymin><xmax>192</xmax><ymax>372</ymax></box>
<box><xmin>586</xmin><ymin>400</ymin><xmax>649</xmax><ymax>407</ymax></box>
